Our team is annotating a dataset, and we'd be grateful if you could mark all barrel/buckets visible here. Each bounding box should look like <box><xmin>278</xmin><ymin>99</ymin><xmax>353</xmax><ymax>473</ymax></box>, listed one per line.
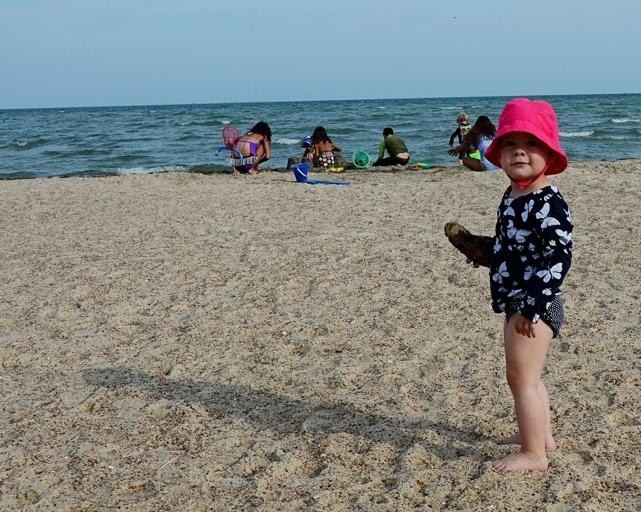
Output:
<box><xmin>290</xmin><ymin>164</ymin><xmax>308</xmax><ymax>182</ymax></box>
<box><xmin>353</xmin><ymin>150</ymin><xmax>370</xmax><ymax>169</ymax></box>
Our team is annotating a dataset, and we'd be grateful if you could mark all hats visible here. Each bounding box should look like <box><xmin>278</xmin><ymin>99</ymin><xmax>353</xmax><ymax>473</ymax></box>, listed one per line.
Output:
<box><xmin>483</xmin><ymin>98</ymin><xmax>568</xmax><ymax>175</ymax></box>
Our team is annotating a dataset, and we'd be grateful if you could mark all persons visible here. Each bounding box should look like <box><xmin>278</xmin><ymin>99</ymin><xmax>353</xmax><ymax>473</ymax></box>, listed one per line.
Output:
<box><xmin>449</xmin><ymin>115</ymin><xmax>500</xmax><ymax>172</ymax></box>
<box><xmin>450</xmin><ymin>112</ymin><xmax>476</xmax><ymax>167</ymax></box>
<box><xmin>372</xmin><ymin>128</ymin><xmax>411</xmax><ymax>167</ymax></box>
<box><xmin>231</xmin><ymin>121</ymin><xmax>272</xmax><ymax>176</ymax></box>
<box><xmin>444</xmin><ymin>96</ymin><xmax>574</xmax><ymax>476</ymax></box>
<box><xmin>311</xmin><ymin>126</ymin><xmax>342</xmax><ymax>169</ymax></box>
